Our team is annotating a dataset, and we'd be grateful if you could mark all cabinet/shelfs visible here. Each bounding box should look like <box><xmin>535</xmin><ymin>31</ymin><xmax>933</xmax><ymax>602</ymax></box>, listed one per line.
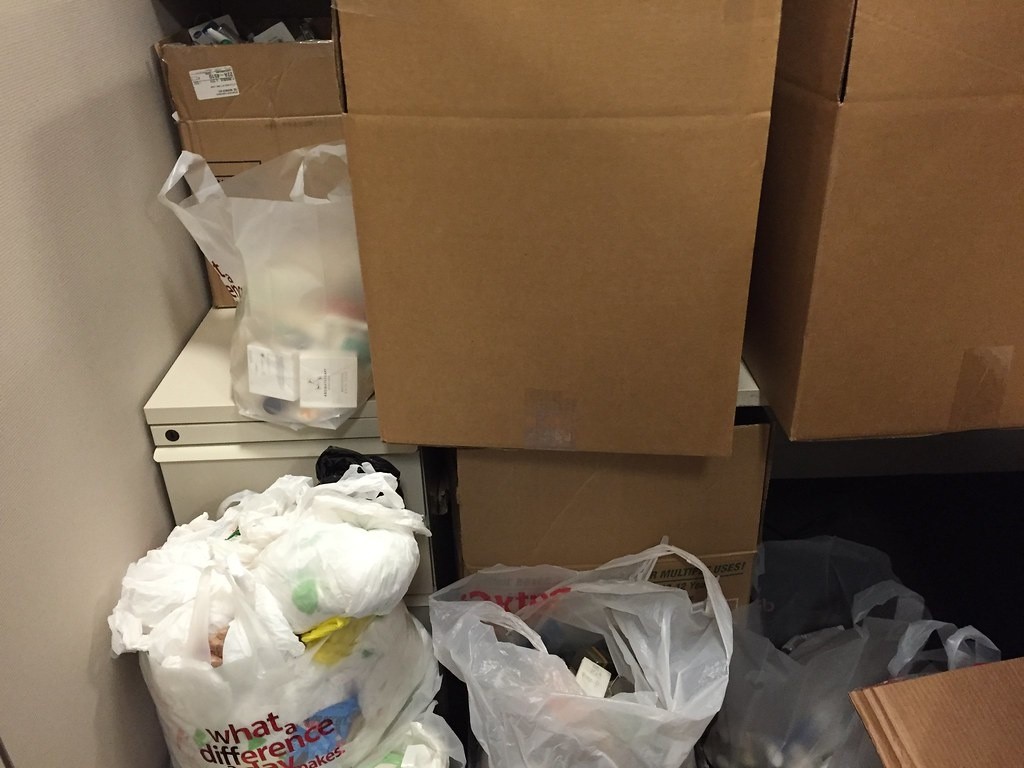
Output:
<box><xmin>142</xmin><ymin>305</ymin><xmax>762</xmax><ymax>637</ymax></box>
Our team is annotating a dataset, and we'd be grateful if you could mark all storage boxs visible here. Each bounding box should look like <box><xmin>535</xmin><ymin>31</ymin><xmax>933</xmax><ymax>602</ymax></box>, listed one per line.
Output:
<box><xmin>455</xmin><ymin>420</ymin><xmax>767</xmax><ymax>630</ymax></box>
<box><xmin>742</xmin><ymin>0</ymin><xmax>1024</xmax><ymax>442</ymax></box>
<box><xmin>151</xmin><ymin>0</ymin><xmax>350</xmax><ymax>310</ymax></box>
<box><xmin>329</xmin><ymin>0</ymin><xmax>786</xmax><ymax>461</ymax></box>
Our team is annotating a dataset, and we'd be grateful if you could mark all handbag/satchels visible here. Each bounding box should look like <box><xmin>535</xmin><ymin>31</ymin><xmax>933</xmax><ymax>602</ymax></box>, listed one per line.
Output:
<box><xmin>705</xmin><ymin>534</ymin><xmax>1000</xmax><ymax>768</ymax></box>
<box><xmin>155</xmin><ymin>142</ymin><xmax>374</xmax><ymax>431</ymax></box>
<box><xmin>428</xmin><ymin>544</ymin><xmax>733</xmax><ymax>768</ymax></box>
<box><xmin>137</xmin><ymin>557</ymin><xmax>467</xmax><ymax>767</ymax></box>
